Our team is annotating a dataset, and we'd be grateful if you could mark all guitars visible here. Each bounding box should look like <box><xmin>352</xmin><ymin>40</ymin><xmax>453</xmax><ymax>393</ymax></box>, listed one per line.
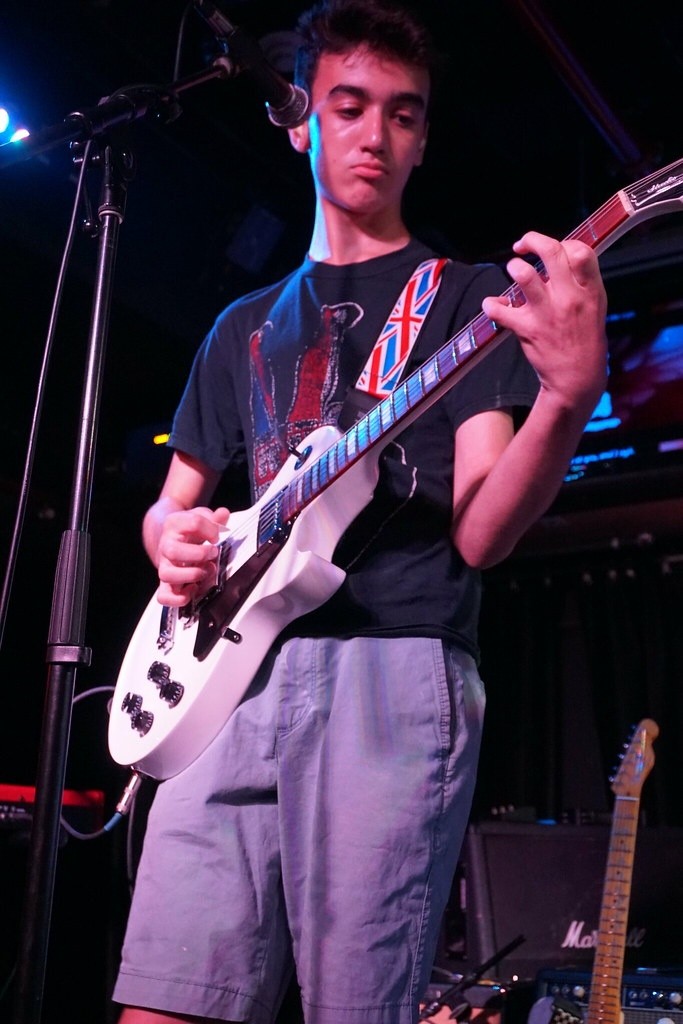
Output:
<box><xmin>524</xmin><ymin>717</ymin><xmax>661</xmax><ymax>1024</ymax></box>
<box><xmin>105</xmin><ymin>156</ymin><xmax>683</xmax><ymax>785</ymax></box>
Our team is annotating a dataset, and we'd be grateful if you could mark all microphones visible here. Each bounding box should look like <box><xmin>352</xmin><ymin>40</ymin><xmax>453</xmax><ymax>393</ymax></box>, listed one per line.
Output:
<box><xmin>192</xmin><ymin>0</ymin><xmax>311</xmax><ymax>128</ymax></box>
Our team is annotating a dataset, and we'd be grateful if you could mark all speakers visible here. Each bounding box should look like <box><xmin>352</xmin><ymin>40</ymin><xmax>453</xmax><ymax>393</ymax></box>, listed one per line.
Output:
<box><xmin>455</xmin><ymin>821</ymin><xmax>683</xmax><ymax>1024</ymax></box>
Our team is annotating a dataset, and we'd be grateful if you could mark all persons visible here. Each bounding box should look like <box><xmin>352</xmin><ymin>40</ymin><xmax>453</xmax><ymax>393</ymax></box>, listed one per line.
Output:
<box><xmin>110</xmin><ymin>0</ymin><xmax>610</xmax><ymax>1024</ymax></box>
